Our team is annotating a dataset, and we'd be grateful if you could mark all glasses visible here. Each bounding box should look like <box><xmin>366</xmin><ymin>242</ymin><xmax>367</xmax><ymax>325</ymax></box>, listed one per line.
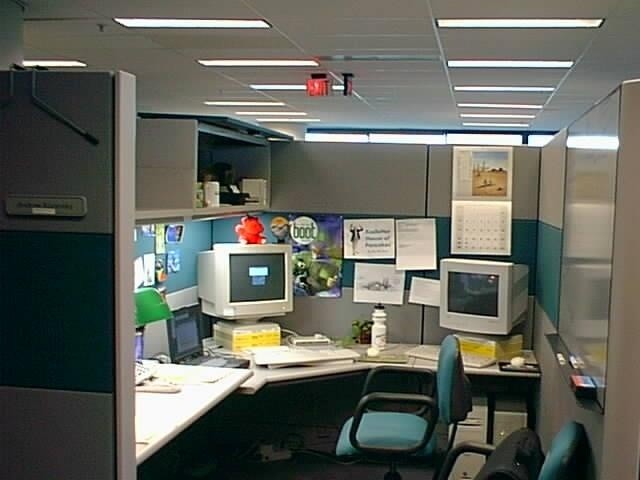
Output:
<box><xmin>272</xmin><ymin>225</ymin><xmax>288</xmax><ymax>229</ymax></box>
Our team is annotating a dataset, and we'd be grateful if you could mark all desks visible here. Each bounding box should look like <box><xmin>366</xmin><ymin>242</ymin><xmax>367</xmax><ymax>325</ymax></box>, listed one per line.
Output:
<box><xmin>241</xmin><ymin>344</ymin><xmax>541</xmax><ymax>463</ymax></box>
<box><xmin>134</xmin><ymin>360</ymin><xmax>255</xmax><ymax>470</ymax></box>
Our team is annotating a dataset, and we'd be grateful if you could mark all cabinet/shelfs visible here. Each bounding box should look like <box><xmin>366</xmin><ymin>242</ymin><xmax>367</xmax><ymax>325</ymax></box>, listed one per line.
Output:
<box><xmin>136</xmin><ymin>116</ymin><xmax>272</xmax><ymax>212</ymax></box>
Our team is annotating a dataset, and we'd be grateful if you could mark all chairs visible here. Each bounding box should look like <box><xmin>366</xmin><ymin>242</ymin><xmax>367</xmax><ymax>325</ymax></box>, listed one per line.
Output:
<box><xmin>335</xmin><ymin>335</ymin><xmax>461</xmax><ymax>480</ymax></box>
<box><xmin>437</xmin><ymin>420</ymin><xmax>584</xmax><ymax>480</ymax></box>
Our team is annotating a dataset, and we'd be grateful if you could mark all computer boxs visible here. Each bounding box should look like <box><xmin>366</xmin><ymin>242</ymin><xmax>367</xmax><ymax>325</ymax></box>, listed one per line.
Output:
<box><xmin>493</xmin><ymin>401</ymin><xmax>528</xmax><ymax>448</ymax></box>
<box><xmin>447</xmin><ymin>394</ymin><xmax>488</xmax><ymax>479</ymax></box>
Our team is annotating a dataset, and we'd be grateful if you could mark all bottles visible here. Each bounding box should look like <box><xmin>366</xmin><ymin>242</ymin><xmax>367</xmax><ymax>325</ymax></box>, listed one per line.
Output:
<box><xmin>369</xmin><ymin>302</ymin><xmax>388</xmax><ymax>350</ymax></box>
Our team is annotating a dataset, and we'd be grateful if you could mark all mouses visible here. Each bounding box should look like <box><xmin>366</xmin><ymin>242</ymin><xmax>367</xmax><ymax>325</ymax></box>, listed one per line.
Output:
<box><xmin>365</xmin><ymin>347</ymin><xmax>380</xmax><ymax>358</ymax></box>
<box><xmin>508</xmin><ymin>355</ymin><xmax>527</xmax><ymax>367</ymax></box>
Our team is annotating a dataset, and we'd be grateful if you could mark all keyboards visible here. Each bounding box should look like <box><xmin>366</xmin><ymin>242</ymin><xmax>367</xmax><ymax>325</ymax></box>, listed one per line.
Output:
<box><xmin>254</xmin><ymin>347</ymin><xmax>360</xmax><ymax>365</ymax></box>
<box><xmin>404</xmin><ymin>344</ymin><xmax>495</xmax><ymax>368</ymax></box>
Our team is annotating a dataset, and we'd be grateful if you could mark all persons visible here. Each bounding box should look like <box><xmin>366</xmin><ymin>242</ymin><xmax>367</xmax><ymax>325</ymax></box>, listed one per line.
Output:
<box><xmin>464</xmin><ymin>275</ymin><xmax>497</xmax><ymax>317</ymax></box>
<box><xmin>349</xmin><ymin>223</ymin><xmax>364</xmax><ymax>256</ymax></box>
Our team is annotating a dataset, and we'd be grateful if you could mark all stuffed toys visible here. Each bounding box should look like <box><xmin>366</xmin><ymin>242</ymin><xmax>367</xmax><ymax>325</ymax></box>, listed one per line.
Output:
<box><xmin>236</xmin><ymin>214</ymin><xmax>268</xmax><ymax>245</ymax></box>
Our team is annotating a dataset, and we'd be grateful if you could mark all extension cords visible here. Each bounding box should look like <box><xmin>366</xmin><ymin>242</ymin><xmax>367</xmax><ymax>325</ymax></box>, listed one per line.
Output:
<box><xmin>257</xmin><ymin>446</ymin><xmax>293</xmax><ymax>462</ymax></box>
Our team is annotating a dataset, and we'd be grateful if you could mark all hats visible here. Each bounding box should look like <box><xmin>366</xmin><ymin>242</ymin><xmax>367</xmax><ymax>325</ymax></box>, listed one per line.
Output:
<box><xmin>271</xmin><ymin>217</ymin><xmax>288</xmax><ymax>225</ymax></box>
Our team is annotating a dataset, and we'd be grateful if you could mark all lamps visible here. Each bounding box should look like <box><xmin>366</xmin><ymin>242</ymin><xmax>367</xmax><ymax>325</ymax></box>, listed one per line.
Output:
<box><xmin>135</xmin><ymin>288</ymin><xmax>174</xmax><ymax>364</ymax></box>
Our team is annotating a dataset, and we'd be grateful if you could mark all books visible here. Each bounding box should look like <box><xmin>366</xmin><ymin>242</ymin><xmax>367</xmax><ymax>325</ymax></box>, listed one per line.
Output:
<box><xmin>241</xmin><ymin>178</ymin><xmax>268</xmax><ymax>208</ymax></box>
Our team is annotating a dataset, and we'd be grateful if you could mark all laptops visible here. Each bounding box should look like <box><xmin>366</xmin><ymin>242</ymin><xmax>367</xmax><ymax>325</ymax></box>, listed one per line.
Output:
<box><xmin>166</xmin><ymin>302</ymin><xmax>250</xmax><ymax>369</ymax></box>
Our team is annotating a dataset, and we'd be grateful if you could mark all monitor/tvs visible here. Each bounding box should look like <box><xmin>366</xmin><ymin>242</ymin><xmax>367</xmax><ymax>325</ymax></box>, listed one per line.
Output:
<box><xmin>196</xmin><ymin>243</ymin><xmax>295</xmax><ymax>351</ymax></box>
<box><xmin>438</xmin><ymin>258</ymin><xmax>529</xmax><ymax>361</ymax></box>
